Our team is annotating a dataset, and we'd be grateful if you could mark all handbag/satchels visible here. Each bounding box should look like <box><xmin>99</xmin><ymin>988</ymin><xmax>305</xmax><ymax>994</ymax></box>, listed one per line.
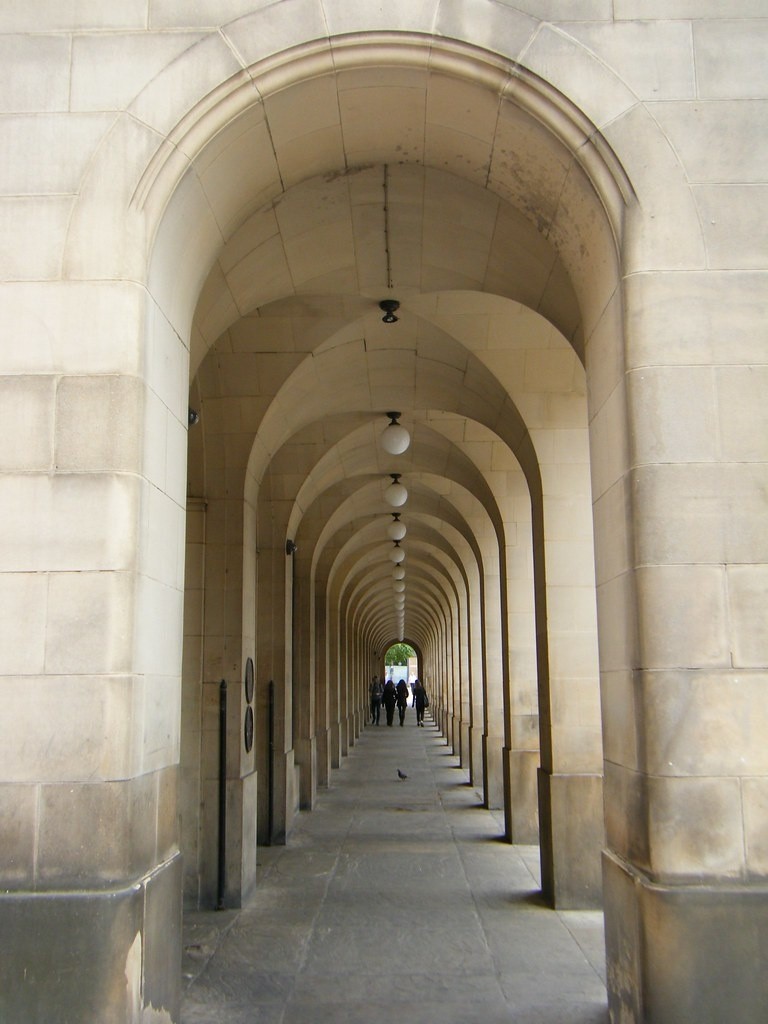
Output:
<box><xmin>424</xmin><ymin>695</ymin><xmax>429</xmax><ymax>707</ymax></box>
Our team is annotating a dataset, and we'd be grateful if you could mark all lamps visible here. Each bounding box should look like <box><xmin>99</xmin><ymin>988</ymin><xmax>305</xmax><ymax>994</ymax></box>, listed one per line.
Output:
<box><xmin>392</xmin><ymin>562</ymin><xmax>405</xmax><ymax>642</ymax></box>
<box><xmin>383</xmin><ymin>473</ymin><xmax>408</xmax><ymax>506</ymax></box>
<box><xmin>380</xmin><ymin>412</ymin><xmax>411</xmax><ymax>455</ymax></box>
<box><xmin>389</xmin><ymin>539</ymin><xmax>405</xmax><ymax>562</ymax></box>
<box><xmin>387</xmin><ymin>513</ymin><xmax>406</xmax><ymax>541</ymax></box>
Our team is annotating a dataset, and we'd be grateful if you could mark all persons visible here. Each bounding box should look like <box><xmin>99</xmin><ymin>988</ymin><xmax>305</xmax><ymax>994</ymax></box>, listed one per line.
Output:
<box><xmin>369</xmin><ymin>675</ymin><xmax>408</xmax><ymax>727</ymax></box>
<box><xmin>412</xmin><ymin>679</ymin><xmax>426</xmax><ymax>727</ymax></box>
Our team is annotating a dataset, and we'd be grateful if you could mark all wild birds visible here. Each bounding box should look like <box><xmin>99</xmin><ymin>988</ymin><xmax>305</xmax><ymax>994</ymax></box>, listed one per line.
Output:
<box><xmin>396</xmin><ymin>769</ymin><xmax>408</xmax><ymax>782</ymax></box>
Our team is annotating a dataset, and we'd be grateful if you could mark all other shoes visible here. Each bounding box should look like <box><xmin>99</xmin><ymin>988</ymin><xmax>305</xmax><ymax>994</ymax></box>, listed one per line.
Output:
<box><xmin>421</xmin><ymin>722</ymin><xmax>424</xmax><ymax>727</ymax></box>
<box><xmin>376</xmin><ymin>720</ymin><xmax>379</xmax><ymax>725</ymax></box>
<box><xmin>400</xmin><ymin>723</ymin><xmax>403</xmax><ymax>727</ymax></box>
<box><xmin>372</xmin><ymin>718</ymin><xmax>375</xmax><ymax>724</ymax></box>
<box><xmin>417</xmin><ymin>722</ymin><xmax>419</xmax><ymax>726</ymax></box>
<box><xmin>387</xmin><ymin>723</ymin><xmax>392</xmax><ymax>726</ymax></box>
